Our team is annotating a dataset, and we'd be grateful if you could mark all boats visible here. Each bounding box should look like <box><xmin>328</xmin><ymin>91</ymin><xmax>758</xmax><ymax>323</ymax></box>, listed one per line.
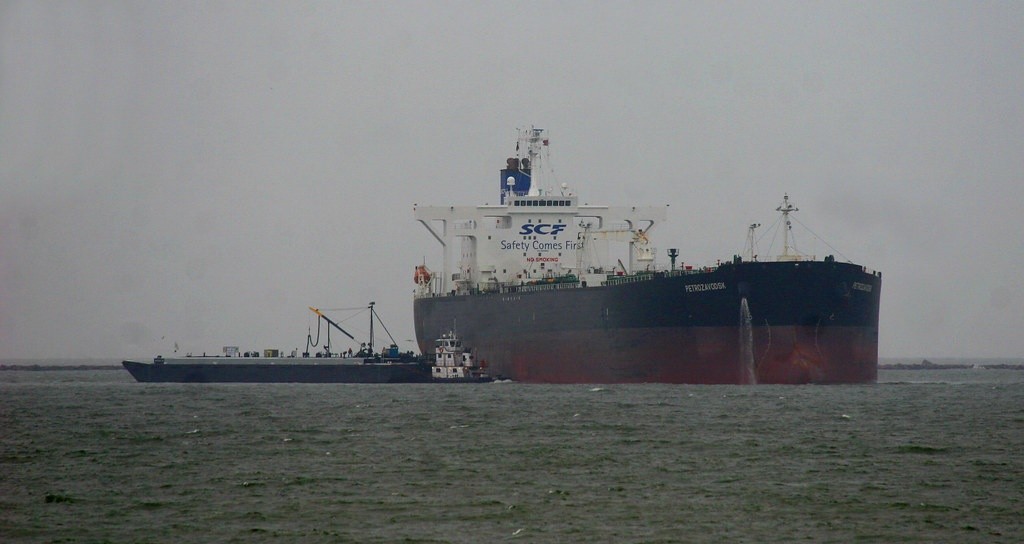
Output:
<box><xmin>122</xmin><ymin>301</ymin><xmax>420</xmax><ymax>384</ymax></box>
<box><xmin>414</xmin><ymin>264</ymin><xmax>431</xmax><ymax>285</ymax></box>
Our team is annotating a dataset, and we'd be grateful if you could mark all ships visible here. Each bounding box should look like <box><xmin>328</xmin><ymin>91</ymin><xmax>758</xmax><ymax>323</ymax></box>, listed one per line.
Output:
<box><xmin>410</xmin><ymin>124</ymin><xmax>884</xmax><ymax>386</ymax></box>
